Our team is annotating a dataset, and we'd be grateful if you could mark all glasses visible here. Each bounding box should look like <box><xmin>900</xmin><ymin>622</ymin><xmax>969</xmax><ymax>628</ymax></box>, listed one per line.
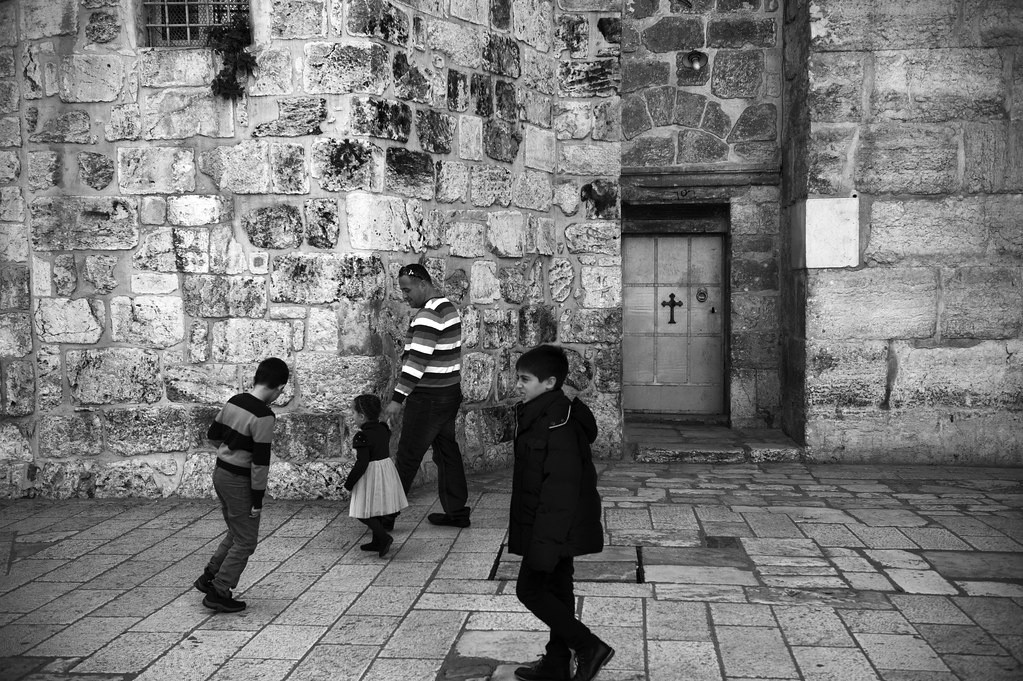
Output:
<box><xmin>399</xmin><ymin>266</ymin><xmax>423</xmax><ymax>279</ymax></box>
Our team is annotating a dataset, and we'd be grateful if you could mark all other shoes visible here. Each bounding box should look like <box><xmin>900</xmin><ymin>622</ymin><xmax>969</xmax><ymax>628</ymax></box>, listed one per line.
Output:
<box><xmin>428</xmin><ymin>506</ymin><xmax>471</xmax><ymax>527</ymax></box>
<box><xmin>194</xmin><ymin>574</ymin><xmax>232</xmax><ymax>597</ymax></box>
<box><xmin>571</xmin><ymin>633</ymin><xmax>616</xmax><ymax>680</ymax></box>
<box><xmin>514</xmin><ymin>653</ymin><xmax>572</xmax><ymax>681</ymax></box>
<box><xmin>384</xmin><ymin>522</ymin><xmax>393</xmax><ymax>531</ymax></box>
<box><xmin>379</xmin><ymin>534</ymin><xmax>394</xmax><ymax>557</ymax></box>
<box><xmin>202</xmin><ymin>592</ymin><xmax>246</xmax><ymax>611</ymax></box>
<box><xmin>360</xmin><ymin>541</ymin><xmax>379</xmax><ymax>551</ymax></box>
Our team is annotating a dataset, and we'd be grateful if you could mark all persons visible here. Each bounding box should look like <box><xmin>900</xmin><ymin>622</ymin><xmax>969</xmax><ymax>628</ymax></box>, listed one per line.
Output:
<box><xmin>385</xmin><ymin>263</ymin><xmax>471</xmax><ymax>528</ymax></box>
<box><xmin>341</xmin><ymin>394</ymin><xmax>408</xmax><ymax>558</ymax></box>
<box><xmin>193</xmin><ymin>357</ymin><xmax>290</xmax><ymax>613</ymax></box>
<box><xmin>507</xmin><ymin>344</ymin><xmax>615</xmax><ymax>681</ymax></box>
